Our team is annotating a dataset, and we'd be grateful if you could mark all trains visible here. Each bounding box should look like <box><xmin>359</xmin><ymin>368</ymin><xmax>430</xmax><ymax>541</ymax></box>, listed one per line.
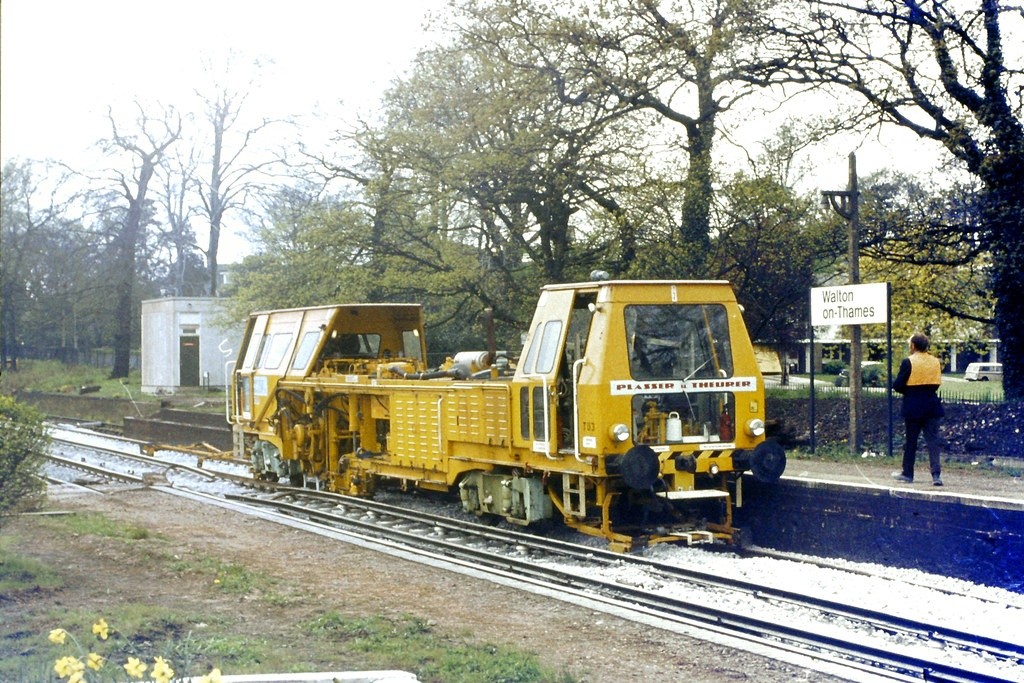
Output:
<box><xmin>223</xmin><ymin>267</ymin><xmax>810</xmax><ymax>558</ymax></box>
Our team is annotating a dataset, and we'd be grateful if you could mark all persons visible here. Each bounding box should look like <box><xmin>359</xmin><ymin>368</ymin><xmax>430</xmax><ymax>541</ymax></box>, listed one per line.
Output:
<box><xmin>893</xmin><ymin>335</ymin><xmax>944</xmax><ymax>486</ymax></box>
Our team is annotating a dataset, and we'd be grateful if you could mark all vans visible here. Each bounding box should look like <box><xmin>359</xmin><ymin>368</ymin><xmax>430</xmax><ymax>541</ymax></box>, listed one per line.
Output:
<box><xmin>965</xmin><ymin>362</ymin><xmax>1004</xmax><ymax>382</ymax></box>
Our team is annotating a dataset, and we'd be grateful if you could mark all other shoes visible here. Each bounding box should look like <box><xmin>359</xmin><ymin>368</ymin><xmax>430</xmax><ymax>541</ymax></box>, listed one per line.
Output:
<box><xmin>892</xmin><ymin>471</ymin><xmax>911</xmax><ymax>482</ymax></box>
<box><xmin>932</xmin><ymin>478</ymin><xmax>943</xmax><ymax>485</ymax></box>
